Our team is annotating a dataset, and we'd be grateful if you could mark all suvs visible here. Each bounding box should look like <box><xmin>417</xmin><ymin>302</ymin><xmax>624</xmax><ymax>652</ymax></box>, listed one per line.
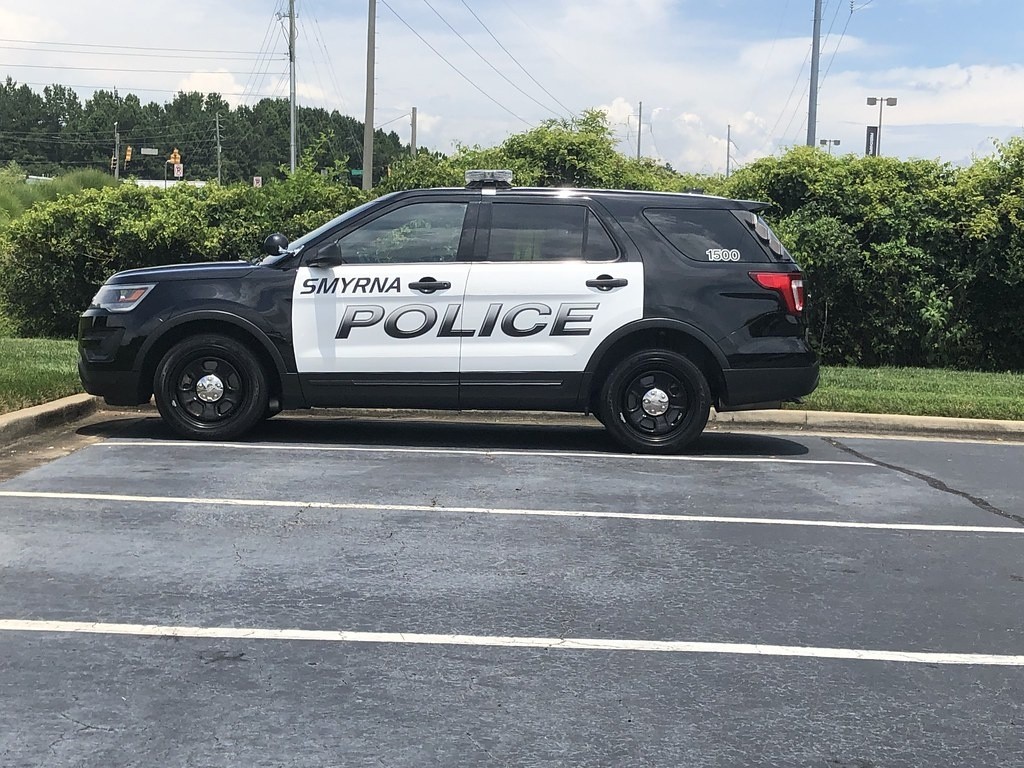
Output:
<box><xmin>73</xmin><ymin>168</ymin><xmax>820</xmax><ymax>456</ymax></box>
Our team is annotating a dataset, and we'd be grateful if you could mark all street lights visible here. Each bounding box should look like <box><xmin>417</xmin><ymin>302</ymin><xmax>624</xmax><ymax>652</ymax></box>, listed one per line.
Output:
<box><xmin>867</xmin><ymin>97</ymin><xmax>898</xmax><ymax>157</ymax></box>
<box><xmin>821</xmin><ymin>138</ymin><xmax>840</xmax><ymax>156</ymax></box>
<box><xmin>163</xmin><ymin>159</ymin><xmax>176</xmax><ymax>196</ymax></box>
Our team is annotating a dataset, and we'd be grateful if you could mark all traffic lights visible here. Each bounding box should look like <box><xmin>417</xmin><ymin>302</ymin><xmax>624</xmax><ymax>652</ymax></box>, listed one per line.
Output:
<box><xmin>175</xmin><ymin>153</ymin><xmax>181</xmax><ymax>166</ymax></box>
<box><xmin>125</xmin><ymin>147</ymin><xmax>132</xmax><ymax>161</ymax></box>
<box><xmin>170</xmin><ymin>154</ymin><xmax>175</xmax><ymax>164</ymax></box>
<box><xmin>110</xmin><ymin>156</ymin><xmax>118</xmax><ymax>171</ymax></box>
